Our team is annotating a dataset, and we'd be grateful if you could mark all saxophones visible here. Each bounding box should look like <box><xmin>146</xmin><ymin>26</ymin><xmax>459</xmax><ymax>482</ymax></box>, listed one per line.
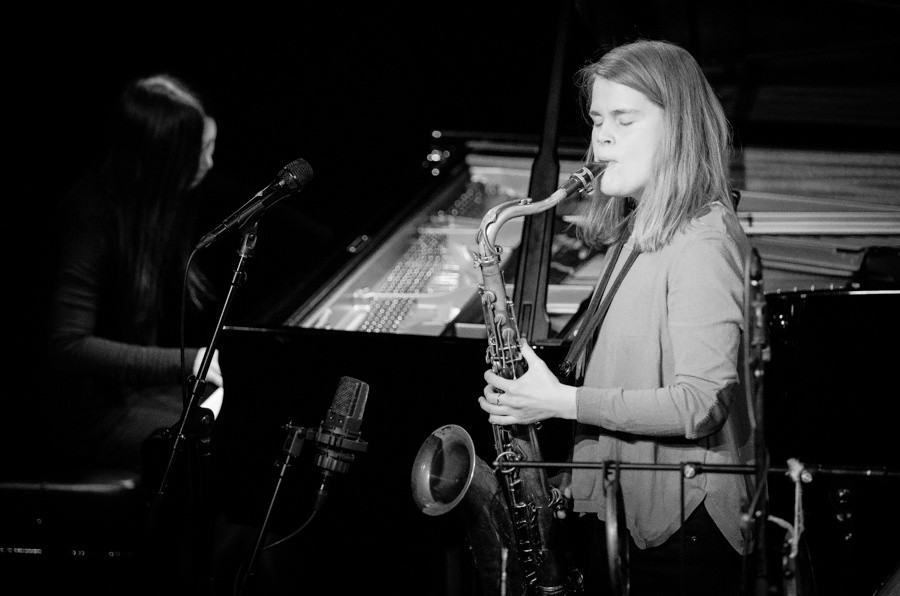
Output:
<box><xmin>409</xmin><ymin>155</ymin><xmax>609</xmax><ymax>596</ymax></box>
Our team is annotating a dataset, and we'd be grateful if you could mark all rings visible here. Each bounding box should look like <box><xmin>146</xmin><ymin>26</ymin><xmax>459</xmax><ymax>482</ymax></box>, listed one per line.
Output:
<box><xmin>497</xmin><ymin>393</ymin><xmax>501</xmax><ymax>406</ymax></box>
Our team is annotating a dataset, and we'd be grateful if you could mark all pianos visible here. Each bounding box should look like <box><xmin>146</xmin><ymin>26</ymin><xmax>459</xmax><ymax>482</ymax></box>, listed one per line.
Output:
<box><xmin>154</xmin><ymin>138</ymin><xmax>899</xmax><ymax>595</ymax></box>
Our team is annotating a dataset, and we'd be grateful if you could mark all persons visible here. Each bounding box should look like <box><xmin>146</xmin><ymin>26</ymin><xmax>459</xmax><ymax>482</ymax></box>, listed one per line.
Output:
<box><xmin>478</xmin><ymin>41</ymin><xmax>755</xmax><ymax>596</ymax></box>
<box><xmin>48</xmin><ymin>73</ymin><xmax>224</xmax><ymax>473</ymax></box>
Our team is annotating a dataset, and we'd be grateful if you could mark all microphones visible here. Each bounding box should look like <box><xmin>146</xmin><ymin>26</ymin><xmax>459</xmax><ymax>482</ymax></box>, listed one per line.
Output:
<box><xmin>195</xmin><ymin>159</ymin><xmax>314</xmax><ymax>250</ymax></box>
<box><xmin>312</xmin><ymin>376</ymin><xmax>370</xmax><ymax>510</ymax></box>
